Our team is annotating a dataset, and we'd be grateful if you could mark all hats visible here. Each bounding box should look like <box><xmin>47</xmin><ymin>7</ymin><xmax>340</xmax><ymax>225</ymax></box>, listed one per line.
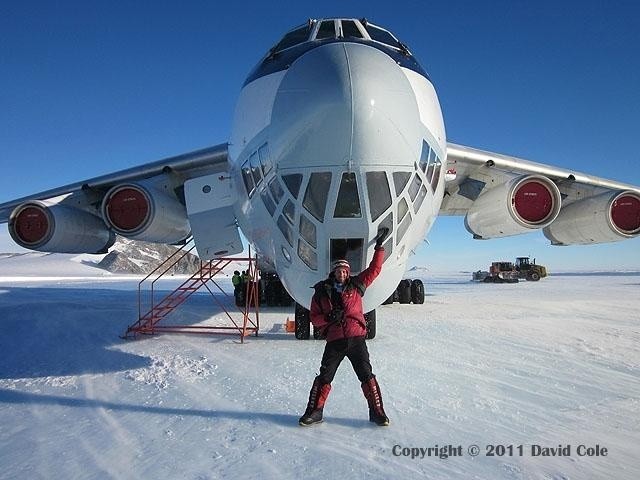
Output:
<box><xmin>331</xmin><ymin>259</ymin><xmax>350</xmax><ymax>270</ymax></box>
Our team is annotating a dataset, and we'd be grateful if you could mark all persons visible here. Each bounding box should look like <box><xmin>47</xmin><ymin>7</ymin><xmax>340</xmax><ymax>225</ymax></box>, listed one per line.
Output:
<box><xmin>299</xmin><ymin>228</ymin><xmax>389</xmax><ymax>425</ymax></box>
<box><xmin>232</xmin><ymin>270</ymin><xmax>254</xmax><ymax>307</ymax></box>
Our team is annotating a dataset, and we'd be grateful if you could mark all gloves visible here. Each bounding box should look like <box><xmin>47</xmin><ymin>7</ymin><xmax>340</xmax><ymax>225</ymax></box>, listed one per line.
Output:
<box><xmin>376</xmin><ymin>227</ymin><xmax>389</xmax><ymax>245</ymax></box>
<box><xmin>324</xmin><ymin>310</ymin><xmax>345</xmax><ymax>322</ymax></box>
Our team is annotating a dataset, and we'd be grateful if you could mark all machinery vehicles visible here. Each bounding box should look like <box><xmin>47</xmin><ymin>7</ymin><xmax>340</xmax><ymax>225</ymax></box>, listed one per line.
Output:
<box><xmin>486</xmin><ymin>256</ymin><xmax>546</xmax><ymax>283</ymax></box>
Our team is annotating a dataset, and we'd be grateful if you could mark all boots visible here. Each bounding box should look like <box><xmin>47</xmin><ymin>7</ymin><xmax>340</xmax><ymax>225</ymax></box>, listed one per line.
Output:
<box><xmin>361</xmin><ymin>376</ymin><xmax>389</xmax><ymax>426</ymax></box>
<box><xmin>299</xmin><ymin>376</ymin><xmax>331</xmax><ymax>426</ymax></box>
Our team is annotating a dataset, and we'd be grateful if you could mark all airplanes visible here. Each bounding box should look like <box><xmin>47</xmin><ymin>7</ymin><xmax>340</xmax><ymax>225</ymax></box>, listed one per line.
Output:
<box><xmin>0</xmin><ymin>17</ymin><xmax>639</xmax><ymax>340</ymax></box>
